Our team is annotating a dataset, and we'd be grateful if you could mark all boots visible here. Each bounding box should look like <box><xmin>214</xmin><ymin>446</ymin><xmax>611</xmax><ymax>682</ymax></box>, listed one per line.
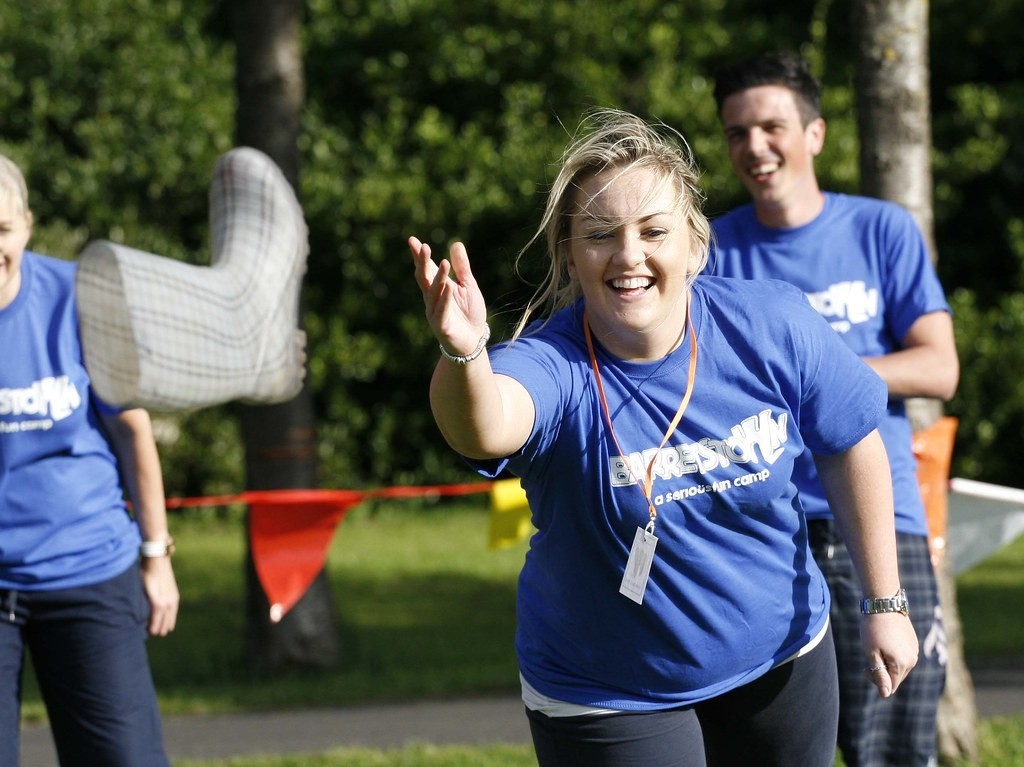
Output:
<box><xmin>73</xmin><ymin>146</ymin><xmax>311</xmax><ymax>415</ymax></box>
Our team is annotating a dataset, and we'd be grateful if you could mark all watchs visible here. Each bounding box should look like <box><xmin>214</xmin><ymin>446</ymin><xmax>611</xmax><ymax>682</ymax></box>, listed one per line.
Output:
<box><xmin>859</xmin><ymin>588</ymin><xmax>910</xmax><ymax>617</ymax></box>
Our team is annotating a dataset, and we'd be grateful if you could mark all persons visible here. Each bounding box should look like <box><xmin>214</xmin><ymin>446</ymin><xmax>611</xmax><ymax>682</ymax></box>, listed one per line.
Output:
<box><xmin>0</xmin><ymin>154</ymin><xmax>181</xmax><ymax>767</ymax></box>
<box><xmin>408</xmin><ymin>50</ymin><xmax>962</xmax><ymax>767</ymax></box>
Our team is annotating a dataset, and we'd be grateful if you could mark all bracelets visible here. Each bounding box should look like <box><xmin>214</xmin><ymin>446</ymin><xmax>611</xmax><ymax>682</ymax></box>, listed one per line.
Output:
<box><xmin>140</xmin><ymin>537</ymin><xmax>176</xmax><ymax>558</ymax></box>
<box><xmin>438</xmin><ymin>322</ymin><xmax>491</xmax><ymax>366</ymax></box>
